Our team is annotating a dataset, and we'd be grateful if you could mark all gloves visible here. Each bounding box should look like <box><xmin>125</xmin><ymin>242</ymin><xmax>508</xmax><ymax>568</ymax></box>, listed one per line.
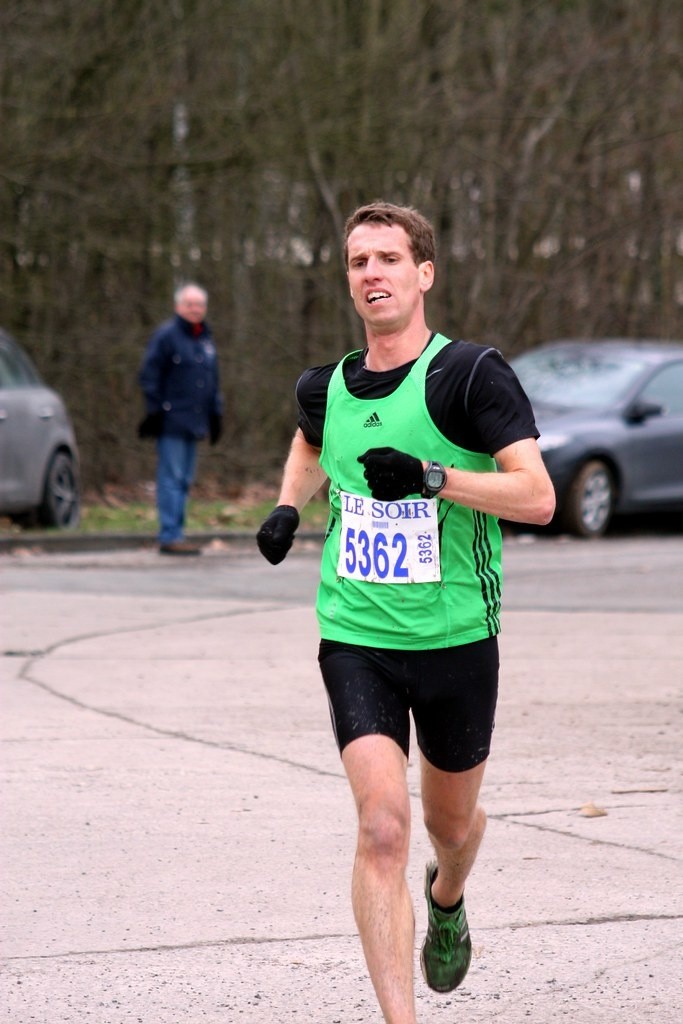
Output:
<box><xmin>357</xmin><ymin>447</ymin><xmax>424</xmax><ymax>502</ymax></box>
<box><xmin>208</xmin><ymin>415</ymin><xmax>223</xmax><ymax>445</ymax></box>
<box><xmin>137</xmin><ymin>413</ymin><xmax>162</xmax><ymax>441</ymax></box>
<box><xmin>255</xmin><ymin>505</ymin><xmax>300</xmax><ymax>565</ymax></box>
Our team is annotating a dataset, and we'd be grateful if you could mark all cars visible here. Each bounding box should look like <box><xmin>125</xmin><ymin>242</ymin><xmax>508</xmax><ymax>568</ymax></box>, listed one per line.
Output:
<box><xmin>0</xmin><ymin>330</ymin><xmax>81</xmax><ymax>531</ymax></box>
<box><xmin>490</xmin><ymin>334</ymin><xmax>683</xmax><ymax>543</ymax></box>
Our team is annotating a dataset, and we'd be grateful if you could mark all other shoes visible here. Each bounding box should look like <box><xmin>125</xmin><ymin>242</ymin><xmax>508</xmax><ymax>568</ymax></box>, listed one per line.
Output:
<box><xmin>160</xmin><ymin>542</ymin><xmax>199</xmax><ymax>556</ymax></box>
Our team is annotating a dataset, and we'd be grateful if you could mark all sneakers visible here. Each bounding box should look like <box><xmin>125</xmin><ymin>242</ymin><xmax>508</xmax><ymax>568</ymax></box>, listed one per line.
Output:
<box><xmin>419</xmin><ymin>860</ymin><xmax>472</xmax><ymax>993</ymax></box>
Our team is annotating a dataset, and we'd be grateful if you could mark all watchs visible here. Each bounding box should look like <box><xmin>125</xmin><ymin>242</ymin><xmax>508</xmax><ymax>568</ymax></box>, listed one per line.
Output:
<box><xmin>421</xmin><ymin>461</ymin><xmax>447</xmax><ymax>499</ymax></box>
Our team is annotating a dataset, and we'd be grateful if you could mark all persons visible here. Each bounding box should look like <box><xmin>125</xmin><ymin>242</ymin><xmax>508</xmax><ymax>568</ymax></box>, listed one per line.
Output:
<box><xmin>135</xmin><ymin>281</ymin><xmax>225</xmax><ymax>555</ymax></box>
<box><xmin>254</xmin><ymin>202</ymin><xmax>556</xmax><ymax>1024</ymax></box>
